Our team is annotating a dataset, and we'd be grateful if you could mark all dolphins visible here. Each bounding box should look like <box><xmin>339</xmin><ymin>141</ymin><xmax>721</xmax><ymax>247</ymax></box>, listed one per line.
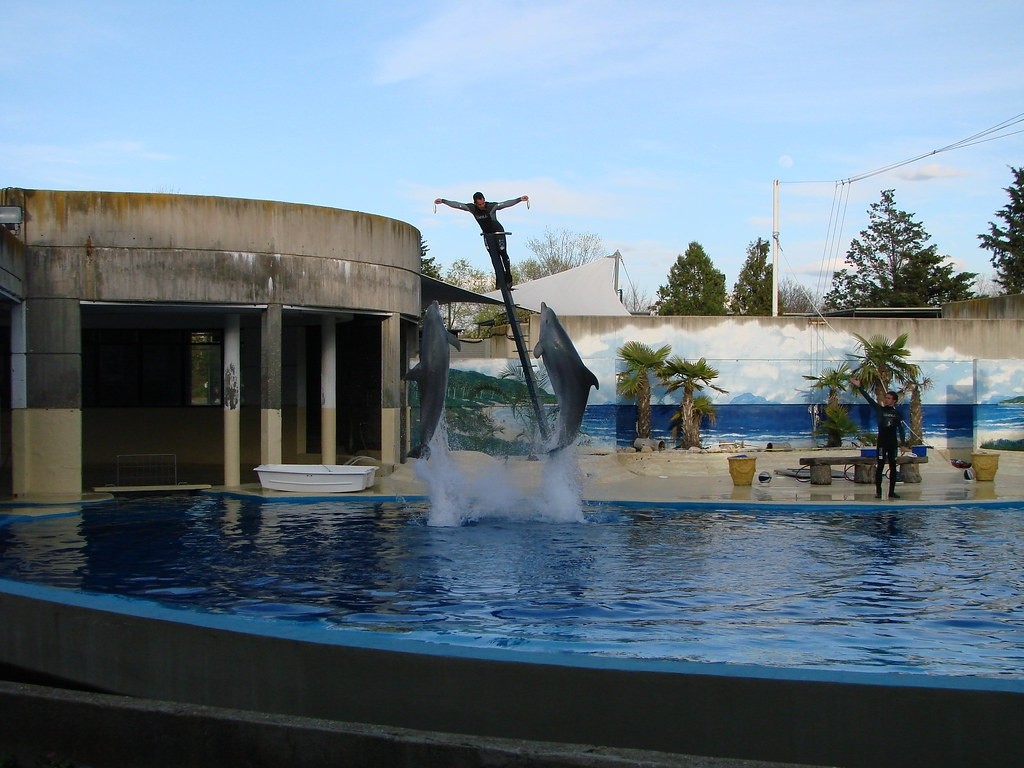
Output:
<box><xmin>403</xmin><ymin>301</ymin><xmax>462</xmax><ymax>463</ymax></box>
<box><xmin>532</xmin><ymin>302</ymin><xmax>600</xmax><ymax>456</ymax></box>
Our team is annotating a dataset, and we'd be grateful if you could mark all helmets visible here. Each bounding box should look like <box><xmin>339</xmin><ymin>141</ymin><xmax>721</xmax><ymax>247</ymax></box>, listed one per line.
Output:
<box><xmin>758</xmin><ymin>472</ymin><xmax>771</xmax><ymax>483</ymax></box>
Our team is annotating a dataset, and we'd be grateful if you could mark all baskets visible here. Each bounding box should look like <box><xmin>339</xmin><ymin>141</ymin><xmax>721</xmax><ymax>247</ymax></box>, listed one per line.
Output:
<box><xmin>971</xmin><ymin>453</ymin><xmax>999</xmax><ymax>481</ymax></box>
<box><xmin>728</xmin><ymin>455</ymin><xmax>756</xmax><ymax>485</ymax></box>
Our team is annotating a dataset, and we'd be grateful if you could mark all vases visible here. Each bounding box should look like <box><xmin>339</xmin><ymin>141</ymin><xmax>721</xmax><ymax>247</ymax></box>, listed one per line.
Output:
<box><xmin>972</xmin><ymin>452</ymin><xmax>1001</xmax><ymax>483</ymax></box>
<box><xmin>726</xmin><ymin>455</ymin><xmax>757</xmax><ymax>487</ymax></box>
<box><xmin>912</xmin><ymin>444</ymin><xmax>927</xmax><ymax>457</ymax></box>
<box><xmin>861</xmin><ymin>448</ymin><xmax>877</xmax><ymax>457</ymax></box>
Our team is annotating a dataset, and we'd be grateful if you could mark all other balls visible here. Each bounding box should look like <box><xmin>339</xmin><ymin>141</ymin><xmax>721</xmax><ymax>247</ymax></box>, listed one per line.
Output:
<box><xmin>759</xmin><ymin>471</ymin><xmax>772</xmax><ymax>483</ymax></box>
<box><xmin>963</xmin><ymin>467</ymin><xmax>976</xmax><ymax>480</ymax></box>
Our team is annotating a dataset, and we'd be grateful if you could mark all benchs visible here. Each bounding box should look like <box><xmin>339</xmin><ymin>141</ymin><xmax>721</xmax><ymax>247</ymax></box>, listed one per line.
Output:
<box><xmin>799</xmin><ymin>456</ymin><xmax>928</xmax><ymax>486</ymax></box>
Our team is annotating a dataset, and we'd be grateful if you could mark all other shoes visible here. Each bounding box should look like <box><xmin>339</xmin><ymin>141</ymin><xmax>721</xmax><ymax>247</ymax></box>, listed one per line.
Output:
<box><xmin>888</xmin><ymin>491</ymin><xmax>900</xmax><ymax>498</ymax></box>
<box><xmin>875</xmin><ymin>492</ymin><xmax>882</xmax><ymax>498</ymax></box>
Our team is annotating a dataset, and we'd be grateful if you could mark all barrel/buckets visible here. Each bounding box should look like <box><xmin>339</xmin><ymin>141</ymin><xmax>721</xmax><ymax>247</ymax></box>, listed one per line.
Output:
<box><xmin>728</xmin><ymin>455</ymin><xmax>758</xmax><ymax>485</ymax></box>
<box><xmin>972</xmin><ymin>453</ymin><xmax>1000</xmax><ymax>481</ymax></box>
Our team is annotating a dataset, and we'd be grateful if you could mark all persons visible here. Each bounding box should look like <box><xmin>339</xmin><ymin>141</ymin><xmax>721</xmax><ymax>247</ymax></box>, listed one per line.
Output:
<box><xmin>435</xmin><ymin>193</ymin><xmax>529</xmax><ymax>291</ymax></box>
<box><xmin>849</xmin><ymin>377</ymin><xmax>905</xmax><ymax>500</ymax></box>
